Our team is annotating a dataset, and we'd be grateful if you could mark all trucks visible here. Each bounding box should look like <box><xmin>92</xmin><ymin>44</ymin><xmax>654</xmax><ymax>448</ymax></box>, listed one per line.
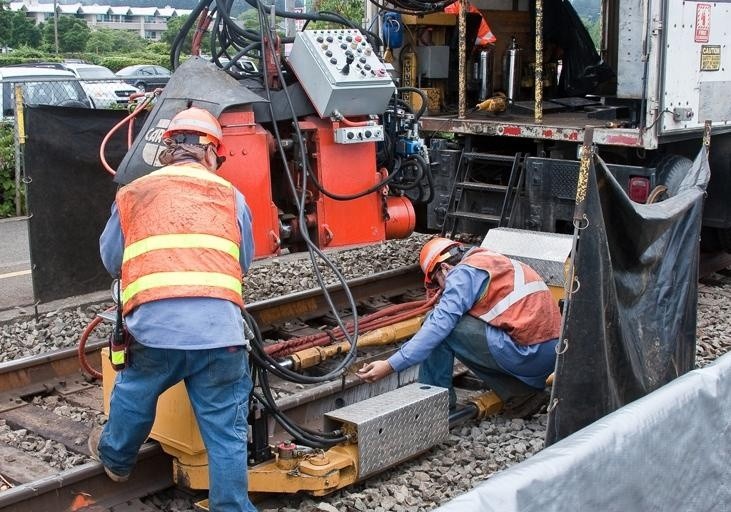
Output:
<box><xmin>365</xmin><ymin>1</ymin><xmax>731</xmax><ymax>226</ymax></box>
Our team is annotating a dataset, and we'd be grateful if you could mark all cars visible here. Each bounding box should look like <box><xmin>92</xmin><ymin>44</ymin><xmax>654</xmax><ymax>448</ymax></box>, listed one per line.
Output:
<box><xmin>115</xmin><ymin>64</ymin><xmax>175</xmax><ymax>92</ymax></box>
<box><xmin>2</xmin><ymin>63</ymin><xmax>146</xmax><ymax>123</ymax></box>
<box><xmin>221</xmin><ymin>60</ymin><xmax>258</xmax><ymax>76</ymax></box>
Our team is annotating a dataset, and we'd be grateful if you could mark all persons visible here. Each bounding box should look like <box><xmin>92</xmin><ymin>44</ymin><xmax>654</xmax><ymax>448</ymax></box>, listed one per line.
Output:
<box><xmin>354</xmin><ymin>235</ymin><xmax>564</xmax><ymax>421</ymax></box>
<box><xmin>85</xmin><ymin>105</ymin><xmax>266</xmax><ymax>512</ymax></box>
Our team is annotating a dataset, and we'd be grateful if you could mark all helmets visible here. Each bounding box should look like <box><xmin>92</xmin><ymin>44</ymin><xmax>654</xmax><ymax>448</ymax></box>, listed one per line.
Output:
<box><xmin>162</xmin><ymin>105</ymin><xmax>224</xmax><ymax>147</ymax></box>
<box><xmin>419</xmin><ymin>236</ymin><xmax>464</xmax><ymax>290</ymax></box>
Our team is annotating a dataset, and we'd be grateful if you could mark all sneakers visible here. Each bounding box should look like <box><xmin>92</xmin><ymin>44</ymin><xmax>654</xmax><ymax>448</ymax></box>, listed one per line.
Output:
<box><xmin>503</xmin><ymin>390</ymin><xmax>549</xmax><ymax>419</ymax></box>
<box><xmin>87</xmin><ymin>425</ymin><xmax>131</xmax><ymax>484</ymax></box>
